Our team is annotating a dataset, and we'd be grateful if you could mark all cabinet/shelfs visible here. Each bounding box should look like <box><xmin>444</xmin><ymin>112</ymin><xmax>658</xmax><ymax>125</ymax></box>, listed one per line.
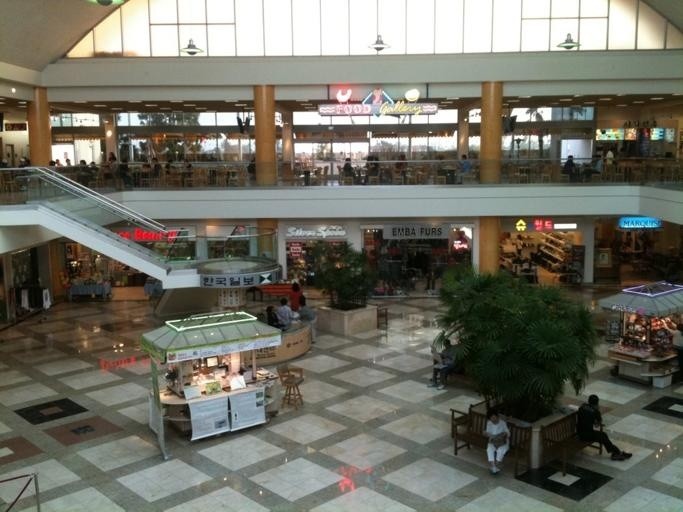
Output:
<box><xmin>538</xmin><ymin>231</ymin><xmax>574</xmax><ymax>273</ymax></box>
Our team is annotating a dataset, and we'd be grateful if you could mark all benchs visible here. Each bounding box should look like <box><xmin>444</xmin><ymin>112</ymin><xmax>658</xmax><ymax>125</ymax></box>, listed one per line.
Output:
<box><xmin>366</xmin><ymin>302</ymin><xmax>388</xmax><ymax>328</ymax></box>
<box><xmin>449</xmin><ymin>395</ymin><xmax>606</xmax><ymax>480</ymax></box>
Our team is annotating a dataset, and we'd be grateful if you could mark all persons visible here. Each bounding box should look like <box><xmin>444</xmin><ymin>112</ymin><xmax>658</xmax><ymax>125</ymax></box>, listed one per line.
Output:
<box><xmin>625</xmin><ymin>242</ymin><xmax>679</xmax><ymax>283</ymax></box>
<box><xmin>362</xmin><ymin>85</ymin><xmax>395</xmax><ymax>104</ymax></box>
<box><xmin>1</xmin><ymin>154</ymin><xmax>31</xmax><ymax>193</ymax></box>
<box><xmin>663</xmin><ymin>319</ymin><xmax>683</xmax><ymax>376</ymax></box>
<box><xmin>268</xmin><ymin>283</ymin><xmax>315</xmax><ymax>324</ymax></box>
<box><xmin>486</xmin><ymin>408</ymin><xmax>510</xmax><ymax>476</ymax></box>
<box><xmin>563</xmin><ymin>154</ymin><xmax>675</xmax><ymax>183</ymax></box>
<box><xmin>577</xmin><ymin>395</ymin><xmax>633</xmax><ymax>462</ymax></box>
<box><xmin>286</xmin><ymin>240</ymin><xmax>335</xmax><ymax>294</ymax></box>
<box><xmin>49</xmin><ymin>153</ymin><xmax>256</xmax><ymax>190</ymax></box>
<box><xmin>520</xmin><ymin>244</ymin><xmax>533</xmax><ymax>268</ymax></box>
<box><xmin>343</xmin><ymin>154</ymin><xmax>473</xmax><ymax>185</ymax></box>
<box><xmin>368</xmin><ymin>250</ymin><xmax>438</xmax><ymax>290</ymax></box>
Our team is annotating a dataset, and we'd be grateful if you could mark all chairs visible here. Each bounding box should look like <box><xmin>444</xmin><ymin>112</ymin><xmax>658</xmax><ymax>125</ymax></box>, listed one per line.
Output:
<box><xmin>277</xmin><ymin>363</ymin><xmax>305</xmax><ymax>410</ymax></box>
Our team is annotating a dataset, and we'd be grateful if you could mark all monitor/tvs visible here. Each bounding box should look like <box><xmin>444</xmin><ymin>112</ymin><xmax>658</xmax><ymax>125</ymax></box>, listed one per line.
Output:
<box><xmin>207</xmin><ymin>356</ymin><xmax>219</xmax><ymax>368</ymax></box>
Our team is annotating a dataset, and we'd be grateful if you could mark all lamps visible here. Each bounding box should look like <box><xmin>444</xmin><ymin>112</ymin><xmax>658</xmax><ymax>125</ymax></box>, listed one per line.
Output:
<box><xmin>179</xmin><ymin>39</ymin><xmax>205</xmax><ymax>56</ymax></box>
<box><xmin>555</xmin><ymin>33</ymin><xmax>582</xmax><ymax>50</ymax></box>
<box><xmin>368</xmin><ymin>35</ymin><xmax>390</xmax><ymax>51</ymax></box>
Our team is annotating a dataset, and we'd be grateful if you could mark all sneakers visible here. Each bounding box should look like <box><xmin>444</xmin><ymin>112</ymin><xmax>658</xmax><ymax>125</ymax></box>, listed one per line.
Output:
<box><xmin>611</xmin><ymin>451</ymin><xmax>632</xmax><ymax>461</ymax></box>
<box><xmin>490</xmin><ymin>466</ymin><xmax>500</xmax><ymax>473</ymax></box>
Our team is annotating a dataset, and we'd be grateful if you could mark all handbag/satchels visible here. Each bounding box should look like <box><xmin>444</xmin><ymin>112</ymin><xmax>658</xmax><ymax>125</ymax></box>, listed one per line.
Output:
<box><xmin>492</xmin><ymin>432</ymin><xmax>506</xmax><ymax>448</ymax></box>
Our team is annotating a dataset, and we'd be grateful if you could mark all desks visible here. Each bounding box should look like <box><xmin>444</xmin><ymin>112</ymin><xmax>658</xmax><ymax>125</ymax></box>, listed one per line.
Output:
<box><xmin>65</xmin><ymin>281</ymin><xmax>112</xmax><ymax>299</ymax></box>
